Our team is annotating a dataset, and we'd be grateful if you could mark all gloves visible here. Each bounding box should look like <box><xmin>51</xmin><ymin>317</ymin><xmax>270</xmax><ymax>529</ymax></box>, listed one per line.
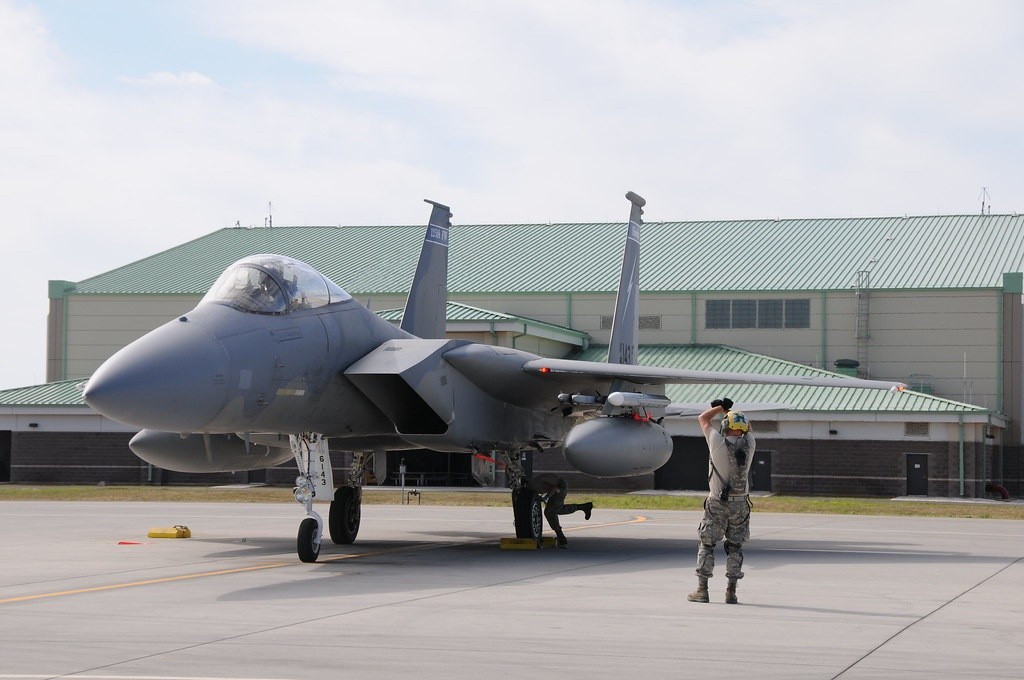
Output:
<box><xmin>710</xmin><ymin>397</ymin><xmax>733</xmax><ymax>412</ymax></box>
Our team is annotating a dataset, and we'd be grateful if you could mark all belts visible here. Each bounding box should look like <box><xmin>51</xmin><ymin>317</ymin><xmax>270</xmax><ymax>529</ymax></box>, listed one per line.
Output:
<box><xmin>709</xmin><ymin>494</ymin><xmax>748</xmax><ymax>502</ymax></box>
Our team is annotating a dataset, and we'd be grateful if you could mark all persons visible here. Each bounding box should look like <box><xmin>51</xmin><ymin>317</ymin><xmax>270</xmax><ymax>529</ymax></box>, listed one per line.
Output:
<box><xmin>538</xmin><ymin>474</ymin><xmax>593</xmax><ymax>545</ymax></box>
<box><xmin>687</xmin><ymin>398</ymin><xmax>756</xmax><ymax>604</ymax></box>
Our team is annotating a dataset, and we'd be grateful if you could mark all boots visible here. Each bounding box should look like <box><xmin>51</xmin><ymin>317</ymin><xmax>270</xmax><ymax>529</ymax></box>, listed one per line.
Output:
<box><xmin>557</xmin><ymin>532</ymin><xmax>567</xmax><ymax>545</ymax></box>
<box><xmin>725</xmin><ymin>587</ymin><xmax>738</xmax><ymax>604</ymax></box>
<box><xmin>687</xmin><ymin>587</ymin><xmax>709</xmax><ymax>602</ymax></box>
<box><xmin>577</xmin><ymin>501</ymin><xmax>593</xmax><ymax>520</ymax></box>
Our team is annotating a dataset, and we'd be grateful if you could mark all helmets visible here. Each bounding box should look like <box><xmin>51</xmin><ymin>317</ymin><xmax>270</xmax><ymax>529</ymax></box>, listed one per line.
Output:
<box><xmin>259</xmin><ymin>264</ymin><xmax>284</xmax><ymax>296</ymax></box>
<box><xmin>721</xmin><ymin>409</ymin><xmax>749</xmax><ymax>433</ymax></box>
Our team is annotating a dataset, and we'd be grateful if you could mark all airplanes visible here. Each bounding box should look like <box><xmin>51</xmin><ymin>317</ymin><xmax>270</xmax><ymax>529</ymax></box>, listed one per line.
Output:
<box><xmin>75</xmin><ymin>192</ymin><xmax>907</xmax><ymax>566</ymax></box>
